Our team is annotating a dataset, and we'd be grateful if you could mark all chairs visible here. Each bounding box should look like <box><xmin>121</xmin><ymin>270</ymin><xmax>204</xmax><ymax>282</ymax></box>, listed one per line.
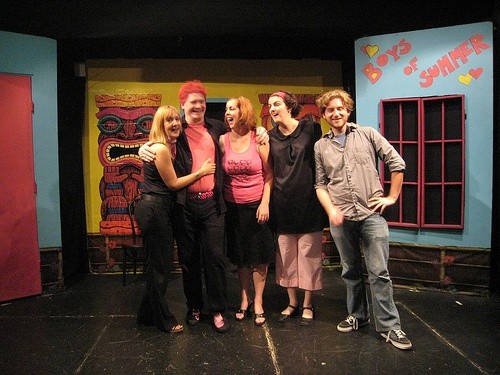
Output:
<box><xmin>121</xmin><ymin>199</ymin><xmax>145</xmax><ymax>286</ymax></box>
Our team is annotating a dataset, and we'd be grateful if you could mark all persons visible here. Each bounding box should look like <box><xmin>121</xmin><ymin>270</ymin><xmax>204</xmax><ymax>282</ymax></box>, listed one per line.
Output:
<box><xmin>314</xmin><ymin>90</ymin><xmax>412</xmax><ymax>349</ymax></box>
<box><xmin>219</xmin><ymin>96</ymin><xmax>273</xmax><ymax>324</ymax></box>
<box><xmin>134</xmin><ymin>105</ymin><xmax>216</xmax><ymax>334</ymax></box>
<box><xmin>138</xmin><ymin>80</ymin><xmax>269</xmax><ymax>331</ymax></box>
<box><xmin>268</xmin><ymin>90</ymin><xmax>323</xmax><ymax>326</ymax></box>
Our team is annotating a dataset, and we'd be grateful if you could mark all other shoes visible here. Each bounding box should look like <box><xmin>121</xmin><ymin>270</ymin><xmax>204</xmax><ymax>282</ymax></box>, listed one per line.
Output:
<box><xmin>277</xmin><ymin>305</ymin><xmax>300</xmax><ymax>320</ymax></box>
<box><xmin>169</xmin><ymin>324</ymin><xmax>183</xmax><ymax>333</ymax></box>
<box><xmin>301</xmin><ymin>307</ymin><xmax>314</xmax><ymax>326</ymax></box>
<box><xmin>191</xmin><ymin>308</ymin><xmax>201</xmax><ymax>320</ymax></box>
<box><xmin>213</xmin><ymin>314</ymin><xmax>224</xmax><ymax>328</ymax></box>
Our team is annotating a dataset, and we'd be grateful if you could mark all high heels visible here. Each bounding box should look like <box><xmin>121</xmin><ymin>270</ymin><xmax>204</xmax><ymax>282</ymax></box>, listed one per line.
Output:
<box><xmin>235</xmin><ymin>303</ymin><xmax>252</xmax><ymax>320</ymax></box>
<box><xmin>254</xmin><ymin>313</ymin><xmax>265</xmax><ymax>326</ymax></box>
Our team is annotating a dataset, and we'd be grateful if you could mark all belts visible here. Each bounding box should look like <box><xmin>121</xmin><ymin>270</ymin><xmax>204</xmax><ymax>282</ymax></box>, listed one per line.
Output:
<box><xmin>187</xmin><ymin>190</ymin><xmax>216</xmax><ymax>201</ymax></box>
<box><xmin>141</xmin><ymin>193</ymin><xmax>174</xmax><ymax>206</ymax></box>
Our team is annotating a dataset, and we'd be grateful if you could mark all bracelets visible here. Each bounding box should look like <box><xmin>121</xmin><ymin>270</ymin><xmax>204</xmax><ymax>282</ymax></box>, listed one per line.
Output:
<box><xmin>195</xmin><ymin>172</ymin><xmax>200</xmax><ymax>178</ymax></box>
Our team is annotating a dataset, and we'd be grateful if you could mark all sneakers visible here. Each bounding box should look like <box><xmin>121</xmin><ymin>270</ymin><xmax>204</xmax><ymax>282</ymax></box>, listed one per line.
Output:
<box><xmin>337</xmin><ymin>315</ymin><xmax>370</xmax><ymax>333</ymax></box>
<box><xmin>380</xmin><ymin>329</ymin><xmax>412</xmax><ymax>350</ymax></box>
